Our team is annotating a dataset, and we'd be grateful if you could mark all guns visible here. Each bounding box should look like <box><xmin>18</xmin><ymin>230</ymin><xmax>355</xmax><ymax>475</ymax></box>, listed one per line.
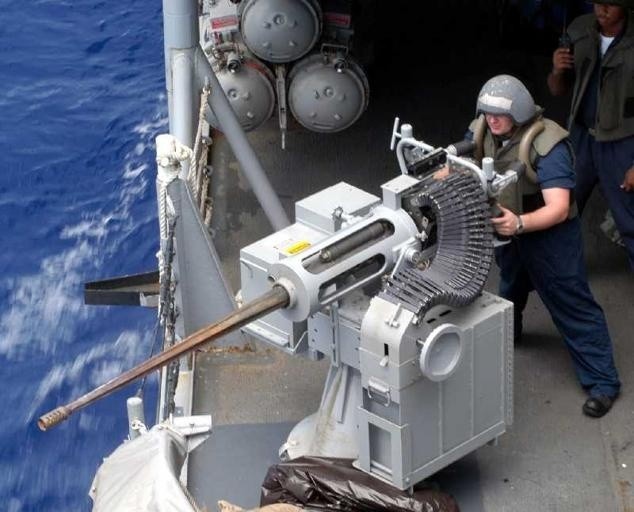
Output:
<box><xmin>37</xmin><ymin>118</ymin><xmax>546</xmax><ymax>498</ymax></box>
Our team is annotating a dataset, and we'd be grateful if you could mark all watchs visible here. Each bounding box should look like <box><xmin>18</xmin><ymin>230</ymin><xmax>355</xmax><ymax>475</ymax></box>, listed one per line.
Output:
<box><xmin>515</xmin><ymin>213</ymin><xmax>523</xmax><ymax>235</ymax></box>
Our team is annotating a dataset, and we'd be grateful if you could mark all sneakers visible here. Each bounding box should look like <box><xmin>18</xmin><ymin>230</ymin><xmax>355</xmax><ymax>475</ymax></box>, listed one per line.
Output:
<box><xmin>583</xmin><ymin>395</ymin><xmax>619</xmax><ymax>418</ymax></box>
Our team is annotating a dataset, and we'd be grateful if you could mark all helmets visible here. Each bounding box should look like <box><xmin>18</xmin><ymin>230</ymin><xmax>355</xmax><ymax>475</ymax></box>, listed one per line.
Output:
<box><xmin>475</xmin><ymin>74</ymin><xmax>536</xmax><ymax>125</ymax></box>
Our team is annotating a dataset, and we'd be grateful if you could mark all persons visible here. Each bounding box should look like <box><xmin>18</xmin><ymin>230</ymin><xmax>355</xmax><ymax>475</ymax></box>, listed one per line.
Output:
<box><xmin>547</xmin><ymin>2</ymin><xmax>634</xmax><ymax>276</ymax></box>
<box><xmin>432</xmin><ymin>75</ymin><xmax>622</xmax><ymax>417</ymax></box>
